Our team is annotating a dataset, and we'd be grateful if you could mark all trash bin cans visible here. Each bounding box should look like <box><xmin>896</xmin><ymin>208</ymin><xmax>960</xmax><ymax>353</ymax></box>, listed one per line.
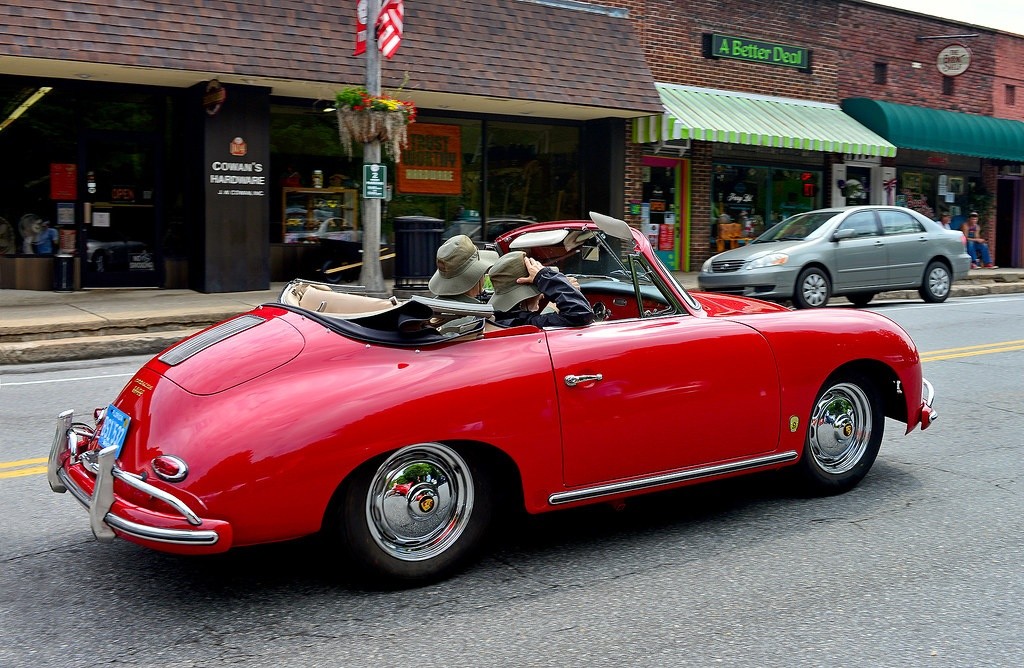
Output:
<box><xmin>394</xmin><ymin>214</ymin><xmax>447</xmax><ymax>289</ymax></box>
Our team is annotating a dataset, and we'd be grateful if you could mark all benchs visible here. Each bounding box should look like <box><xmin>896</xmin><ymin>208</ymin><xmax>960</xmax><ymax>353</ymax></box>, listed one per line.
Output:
<box><xmin>300</xmin><ymin>285</ymin><xmax>511</xmax><ymax>332</ymax></box>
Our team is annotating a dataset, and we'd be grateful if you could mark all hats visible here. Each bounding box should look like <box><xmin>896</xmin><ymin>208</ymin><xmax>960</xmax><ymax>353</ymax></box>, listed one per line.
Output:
<box><xmin>428</xmin><ymin>235</ymin><xmax>499</xmax><ymax>296</ymax></box>
<box><xmin>942</xmin><ymin>212</ymin><xmax>951</xmax><ymax>216</ymax></box>
<box><xmin>486</xmin><ymin>251</ymin><xmax>559</xmax><ymax>313</ymax></box>
<box><xmin>969</xmin><ymin>212</ymin><xmax>979</xmax><ymax>216</ymax></box>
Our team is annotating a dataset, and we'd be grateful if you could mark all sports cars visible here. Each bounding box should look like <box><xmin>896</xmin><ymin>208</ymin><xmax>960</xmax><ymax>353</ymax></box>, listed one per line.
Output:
<box><xmin>44</xmin><ymin>212</ymin><xmax>938</xmax><ymax>585</ymax></box>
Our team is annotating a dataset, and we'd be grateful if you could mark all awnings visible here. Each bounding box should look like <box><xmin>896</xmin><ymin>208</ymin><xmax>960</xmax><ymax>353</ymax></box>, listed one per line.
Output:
<box><xmin>840</xmin><ymin>97</ymin><xmax>1024</xmax><ymax>162</ymax></box>
<box><xmin>632</xmin><ymin>82</ymin><xmax>899</xmax><ymax>157</ymax></box>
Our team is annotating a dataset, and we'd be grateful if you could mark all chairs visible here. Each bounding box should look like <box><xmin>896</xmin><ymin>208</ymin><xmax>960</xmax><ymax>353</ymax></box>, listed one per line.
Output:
<box><xmin>952</xmin><ymin>214</ymin><xmax>965</xmax><ymax>230</ymax></box>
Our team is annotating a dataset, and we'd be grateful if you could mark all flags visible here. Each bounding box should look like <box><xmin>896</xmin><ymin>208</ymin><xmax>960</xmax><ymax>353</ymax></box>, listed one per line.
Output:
<box><xmin>375</xmin><ymin>0</ymin><xmax>403</xmax><ymax>59</ymax></box>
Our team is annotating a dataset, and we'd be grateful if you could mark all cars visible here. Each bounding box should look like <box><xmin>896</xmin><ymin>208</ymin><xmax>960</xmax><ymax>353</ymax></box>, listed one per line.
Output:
<box><xmin>286</xmin><ymin>217</ymin><xmax>362</xmax><ymax>245</ymax></box>
<box><xmin>697</xmin><ymin>204</ymin><xmax>973</xmax><ymax>312</ymax></box>
<box><xmin>440</xmin><ymin>215</ymin><xmax>543</xmax><ymax>262</ymax></box>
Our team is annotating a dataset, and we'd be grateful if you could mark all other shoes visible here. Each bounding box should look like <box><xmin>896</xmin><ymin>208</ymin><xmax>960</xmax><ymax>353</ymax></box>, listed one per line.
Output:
<box><xmin>971</xmin><ymin>263</ymin><xmax>982</xmax><ymax>269</ymax></box>
<box><xmin>983</xmin><ymin>263</ymin><xmax>999</xmax><ymax>269</ymax></box>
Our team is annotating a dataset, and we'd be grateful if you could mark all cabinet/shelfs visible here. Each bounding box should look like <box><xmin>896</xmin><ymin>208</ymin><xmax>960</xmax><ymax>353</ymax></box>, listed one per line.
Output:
<box><xmin>281</xmin><ymin>187</ymin><xmax>357</xmax><ymax>243</ymax></box>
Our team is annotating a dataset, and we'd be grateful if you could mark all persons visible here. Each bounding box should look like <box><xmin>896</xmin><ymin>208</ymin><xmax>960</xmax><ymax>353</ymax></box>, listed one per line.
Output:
<box><xmin>428</xmin><ymin>234</ymin><xmax>594</xmax><ymax>329</ymax></box>
<box><xmin>935</xmin><ymin>211</ymin><xmax>998</xmax><ymax>269</ymax></box>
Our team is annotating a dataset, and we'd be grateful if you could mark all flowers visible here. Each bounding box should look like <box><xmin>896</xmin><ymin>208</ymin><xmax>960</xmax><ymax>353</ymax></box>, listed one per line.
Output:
<box><xmin>334</xmin><ymin>65</ymin><xmax>421</xmax><ymax>163</ymax></box>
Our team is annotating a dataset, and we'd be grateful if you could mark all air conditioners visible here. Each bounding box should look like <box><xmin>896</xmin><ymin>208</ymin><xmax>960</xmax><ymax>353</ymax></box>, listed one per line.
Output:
<box><xmin>1003</xmin><ymin>165</ymin><xmax>1024</xmax><ymax>176</ymax></box>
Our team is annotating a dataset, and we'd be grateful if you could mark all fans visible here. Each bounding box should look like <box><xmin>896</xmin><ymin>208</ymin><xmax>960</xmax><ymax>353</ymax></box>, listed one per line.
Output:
<box><xmin>19</xmin><ymin>214</ymin><xmax>49</xmax><ymax>255</ymax></box>
<box><xmin>0</xmin><ymin>217</ymin><xmax>15</xmax><ymax>255</ymax></box>
